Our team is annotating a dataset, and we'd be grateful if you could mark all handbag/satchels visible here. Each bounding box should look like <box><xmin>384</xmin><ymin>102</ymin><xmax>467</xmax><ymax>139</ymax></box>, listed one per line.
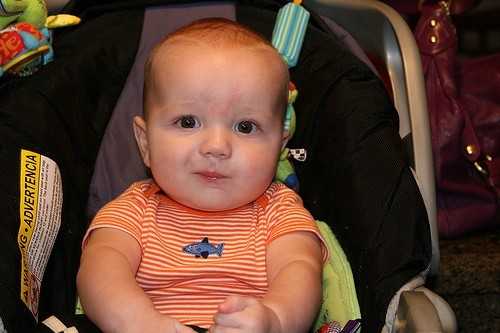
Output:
<box><xmin>414</xmin><ymin>0</ymin><xmax>500</xmax><ymax>240</ymax></box>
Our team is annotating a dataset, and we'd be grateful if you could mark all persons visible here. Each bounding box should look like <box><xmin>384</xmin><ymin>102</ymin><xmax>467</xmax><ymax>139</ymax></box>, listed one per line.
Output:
<box><xmin>76</xmin><ymin>17</ymin><xmax>331</xmax><ymax>333</ymax></box>
